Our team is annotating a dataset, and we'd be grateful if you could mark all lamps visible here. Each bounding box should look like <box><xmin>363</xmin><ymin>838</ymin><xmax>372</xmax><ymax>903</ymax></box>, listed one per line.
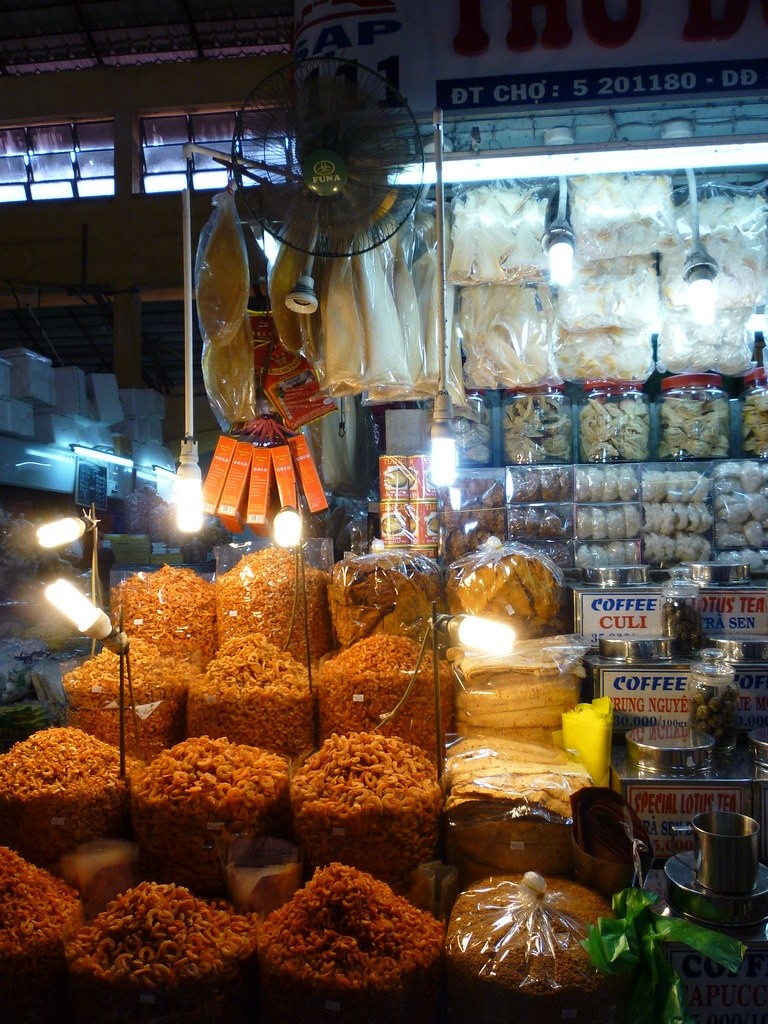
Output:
<box><xmin>35</xmin><ymin>502</ymin><xmax>105</xmax><ymax>659</ymax></box>
<box><xmin>540</xmin><ymin>177</ymin><xmax>577</xmax><ymax>288</ymax></box>
<box><xmin>428</xmin><ymin>106</ymin><xmax>460</xmax><ymax>490</ymax></box>
<box><xmin>174</xmin><ymin>188</ymin><xmax>204</xmax><ymax>533</ymax></box>
<box><xmin>152</xmin><ymin>465</ymin><xmax>176</xmax><ymax>478</ymax></box>
<box><xmin>68</xmin><ymin>444</ymin><xmax>135</xmax><ymax>468</ymax></box>
<box><xmin>426</xmin><ymin>601</ymin><xmax>515</xmax><ymax>781</ymax></box>
<box><xmin>272</xmin><ymin>505</ymin><xmax>315</xmax><ymax>695</ymax></box>
<box><xmin>681</xmin><ymin>168</ymin><xmax>720</xmax><ymax>325</ymax></box>
<box><xmin>45</xmin><ymin>578</ymin><xmax>137</xmax><ymax>777</ymax></box>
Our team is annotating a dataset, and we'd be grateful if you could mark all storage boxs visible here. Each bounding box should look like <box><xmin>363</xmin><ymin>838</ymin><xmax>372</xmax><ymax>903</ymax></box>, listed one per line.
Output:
<box><xmin>0</xmin><ymin>347</ymin><xmax>56</xmax><ymax>408</ymax></box>
<box><xmin>270</xmin><ymin>445</ymin><xmax>301</xmax><ymax>510</ymax></box>
<box><xmin>0</xmin><ymin>396</ymin><xmax>35</xmax><ymax>438</ymax></box>
<box><xmin>131</xmin><ymin>441</ymin><xmax>178</xmax><ymax>472</ymax></box>
<box><xmin>0</xmin><ymin>358</ymin><xmax>13</xmax><ymax>397</ymax></box>
<box><xmin>257</xmin><ymin>342</ymin><xmax>338</xmax><ymax>430</ymax></box>
<box><xmin>144</xmin><ymin>415</ymin><xmax>164</xmax><ymax>446</ymax></box>
<box><xmin>217</xmin><ymin>441</ymin><xmax>254</xmax><ymax>533</ymax></box>
<box><xmin>288</xmin><ymin>434</ymin><xmax>329</xmax><ymax>513</ymax></box>
<box><xmin>112</xmin><ymin>432</ymin><xmax>126</xmax><ymax>456</ymax></box>
<box><xmin>111</xmin><ymin>419</ymin><xmax>147</xmax><ymax>444</ymax></box>
<box><xmin>202</xmin><ymin>436</ymin><xmax>237</xmax><ymax>516</ymax></box>
<box><xmin>85</xmin><ymin>372</ymin><xmax>124</xmax><ymax>427</ymax></box>
<box><xmin>139</xmin><ymin>388</ymin><xmax>166</xmax><ymax>420</ymax></box>
<box><xmin>118</xmin><ymin>389</ymin><xmax>150</xmax><ymax>417</ymax></box>
<box><xmin>245</xmin><ymin>447</ymin><xmax>275</xmax><ymax>537</ymax></box>
<box><xmin>33</xmin><ymin>366</ymin><xmax>88</xmax><ymax>418</ymax></box>
<box><xmin>34</xmin><ymin>413</ymin><xmax>81</xmax><ymax>446</ymax></box>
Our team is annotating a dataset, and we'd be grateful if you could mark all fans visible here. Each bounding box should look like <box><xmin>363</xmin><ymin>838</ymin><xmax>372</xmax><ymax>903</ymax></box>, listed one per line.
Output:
<box><xmin>230</xmin><ymin>55</ymin><xmax>425</xmax><ymax>258</ymax></box>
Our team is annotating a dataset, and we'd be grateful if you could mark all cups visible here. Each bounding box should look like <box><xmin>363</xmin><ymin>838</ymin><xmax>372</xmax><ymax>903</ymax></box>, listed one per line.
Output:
<box><xmin>672</xmin><ymin>812</ymin><xmax>760</xmax><ymax>894</ymax></box>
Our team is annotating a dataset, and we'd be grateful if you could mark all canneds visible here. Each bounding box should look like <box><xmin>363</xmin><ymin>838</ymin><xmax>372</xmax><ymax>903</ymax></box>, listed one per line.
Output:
<box><xmin>378</xmin><ymin>453</ymin><xmax>440</xmax><ymax>573</ymax></box>
